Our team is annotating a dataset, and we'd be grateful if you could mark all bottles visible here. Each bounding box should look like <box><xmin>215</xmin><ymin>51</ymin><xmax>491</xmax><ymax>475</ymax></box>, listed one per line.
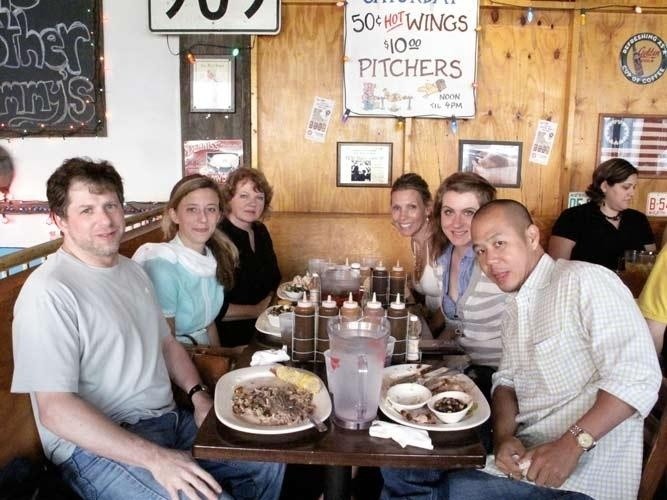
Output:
<box><xmin>363</xmin><ymin>291</ymin><xmax>384</xmax><ymax>326</ymax></box>
<box><xmin>340</xmin><ymin>290</ymin><xmax>360</xmax><ymax>322</ymax></box>
<box><xmin>372</xmin><ymin>260</ymin><xmax>388</xmax><ymax>308</ymax></box>
<box><xmin>388</xmin><ymin>259</ymin><xmax>406</xmax><ymax>304</ymax></box>
<box><xmin>351</xmin><ymin>262</ymin><xmax>360</xmax><ymax>279</ymax></box>
<box><xmin>292</xmin><ymin>290</ymin><xmax>315</xmax><ymax>361</ymax></box>
<box><xmin>406</xmin><ymin>315</ymin><xmax>419</xmax><ymax>362</ymax></box>
<box><xmin>386</xmin><ymin>293</ymin><xmax>409</xmax><ymax>364</ymax></box>
<box><xmin>316</xmin><ymin>295</ymin><xmax>339</xmax><ymax>363</ymax></box>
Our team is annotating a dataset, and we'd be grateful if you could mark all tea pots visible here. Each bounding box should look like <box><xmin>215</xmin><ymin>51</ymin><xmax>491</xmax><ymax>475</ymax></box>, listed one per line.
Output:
<box><xmin>327</xmin><ymin>315</ymin><xmax>391</xmax><ymax>424</ymax></box>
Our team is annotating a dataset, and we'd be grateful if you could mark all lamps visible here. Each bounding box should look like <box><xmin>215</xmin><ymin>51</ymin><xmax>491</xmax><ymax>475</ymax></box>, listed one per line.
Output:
<box><xmin>340</xmin><ymin>54</ymin><xmax>349</xmax><ymax>63</ymax></box>
<box><xmin>395</xmin><ymin>116</ymin><xmax>404</xmax><ymax>131</ymax></box>
<box><xmin>634</xmin><ymin>6</ymin><xmax>642</xmax><ymax>14</ymax></box>
<box><xmin>450</xmin><ymin>115</ymin><xmax>458</xmax><ymax>134</ymax></box>
<box><xmin>580</xmin><ymin>8</ymin><xmax>586</xmax><ymax>25</ymax></box>
<box><xmin>336</xmin><ymin>1</ymin><xmax>348</xmax><ymax>7</ymax></box>
<box><xmin>185</xmin><ymin>51</ymin><xmax>196</xmax><ymax>65</ymax></box>
<box><xmin>230</xmin><ymin>47</ymin><xmax>240</xmax><ymax>57</ymax></box>
<box><xmin>341</xmin><ymin>109</ymin><xmax>351</xmax><ymax>125</ymax></box>
<box><xmin>527</xmin><ymin>7</ymin><xmax>533</xmax><ymax>24</ymax></box>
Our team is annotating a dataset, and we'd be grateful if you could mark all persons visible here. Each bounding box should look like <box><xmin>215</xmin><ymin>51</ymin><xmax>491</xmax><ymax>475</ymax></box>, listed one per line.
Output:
<box><xmin>632</xmin><ymin>242</ymin><xmax>667</xmax><ymax>354</ymax></box>
<box><xmin>10</xmin><ymin>160</ymin><xmax>286</xmax><ymax>500</ymax></box>
<box><xmin>382</xmin><ymin>200</ymin><xmax>663</xmax><ymax>500</ymax></box>
<box><xmin>206</xmin><ymin>168</ymin><xmax>282</xmax><ymax>344</ymax></box>
<box><xmin>132</xmin><ymin>174</ymin><xmax>233</xmax><ymax>346</ymax></box>
<box><xmin>390</xmin><ymin>174</ymin><xmax>444</xmax><ymax>335</ymax></box>
<box><xmin>548</xmin><ymin>158</ymin><xmax>658</xmax><ymax>277</ymax></box>
<box><xmin>429</xmin><ymin>172</ymin><xmax>507</xmax><ymax>385</ymax></box>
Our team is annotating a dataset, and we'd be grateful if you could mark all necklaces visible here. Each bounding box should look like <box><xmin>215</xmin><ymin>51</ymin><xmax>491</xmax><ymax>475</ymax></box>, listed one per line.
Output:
<box><xmin>600</xmin><ymin>210</ymin><xmax>624</xmax><ymax>223</ymax></box>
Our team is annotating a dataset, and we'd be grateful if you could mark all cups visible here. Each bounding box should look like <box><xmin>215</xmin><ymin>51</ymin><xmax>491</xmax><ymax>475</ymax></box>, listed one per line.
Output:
<box><xmin>322</xmin><ymin>351</ymin><xmax>332</xmax><ymax>392</ymax></box>
<box><xmin>616</xmin><ymin>251</ymin><xmax>658</xmax><ymax>299</ymax></box>
<box><xmin>384</xmin><ymin>336</ymin><xmax>396</xmax><ymax>369</ymax></box>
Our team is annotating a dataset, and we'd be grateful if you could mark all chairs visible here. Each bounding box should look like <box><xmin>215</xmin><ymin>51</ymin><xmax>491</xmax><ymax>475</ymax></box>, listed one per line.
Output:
<box><xmin>0</xmin><ymin>300</ymin><xmax>249</xmax><ymax>498</ymax></box>
<box><xmin>636</xmin><ymin>370</ymin><xmax>667</xmax><ymax>497</ymax></box>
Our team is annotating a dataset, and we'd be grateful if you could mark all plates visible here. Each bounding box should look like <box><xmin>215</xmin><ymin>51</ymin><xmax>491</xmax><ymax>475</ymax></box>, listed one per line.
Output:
<box><xmin>214</xmin><ymin>364</ymin><xmax>332</xmax><ymax>435</ymax></box>
<box><xmin>379</xmin><ymin>363</ymin><xmax>491</xmax><ymax>431</ymax></box>
<box><xmin>255</xmin><ymin>304</ymin><xmax>296</xmax><ymax>338</ymax></box>
<box><xmin>277</xmin><ymin>281</ymin><xmax>310</xmax><ymax>302</ymax></box>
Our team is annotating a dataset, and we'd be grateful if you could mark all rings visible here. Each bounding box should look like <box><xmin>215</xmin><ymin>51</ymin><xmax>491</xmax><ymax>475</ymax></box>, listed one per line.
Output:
<box><xmin>506</xmin><ymin>472</ymin><xmax>513</xmax><ymax>481</ymax></box>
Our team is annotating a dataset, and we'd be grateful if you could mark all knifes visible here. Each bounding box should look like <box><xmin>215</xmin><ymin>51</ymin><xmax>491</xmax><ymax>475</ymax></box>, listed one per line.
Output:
<box><xmin>390</xmin><ymin>360</ymin><xmax>449</xmax><ymax>386</ymax></box>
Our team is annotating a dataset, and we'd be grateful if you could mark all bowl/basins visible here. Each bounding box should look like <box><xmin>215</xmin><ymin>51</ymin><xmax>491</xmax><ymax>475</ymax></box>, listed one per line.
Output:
<box><xmin>427</xmin><ymin>391</ymin><xmax>474</xmax><ymax>424</ymax></box>
<box><xmin>388</xmin><ymin>383</ymin><xmax>432</xmax><ymax>411</ymax></box>
<box><xmin>282</xmin><ymin>286</ymin><xmax>305</xmax><ymax>299</ymax></box>
<box><xmin>266</xmin><ymin>306</ymin><xmax>295</xmax><ymax>327</ymax></box>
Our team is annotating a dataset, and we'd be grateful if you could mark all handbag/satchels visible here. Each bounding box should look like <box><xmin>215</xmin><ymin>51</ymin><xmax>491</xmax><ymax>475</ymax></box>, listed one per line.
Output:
<box><xmin>177</xmin><ymin>332</ymin><xmax>230</xmax><ymax>414</ymax></box>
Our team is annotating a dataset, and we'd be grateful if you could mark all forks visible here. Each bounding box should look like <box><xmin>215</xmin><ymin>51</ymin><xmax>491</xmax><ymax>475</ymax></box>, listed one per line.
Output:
<box><xmin>273</xmin><ymin>391</ymin><xmax>328</xmax><ymax>433</ymax></box>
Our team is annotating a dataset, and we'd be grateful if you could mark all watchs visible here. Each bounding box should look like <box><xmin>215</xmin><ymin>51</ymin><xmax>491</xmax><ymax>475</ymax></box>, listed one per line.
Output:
<box><xmin>187</xmin><ymin>383</ymin><xmax>210</xmax><ymax>400</ymax></box>
<box><xmin>568</xmin><ymin>424</ymin><xmax>596</xmax><ymax>452</ymax></box>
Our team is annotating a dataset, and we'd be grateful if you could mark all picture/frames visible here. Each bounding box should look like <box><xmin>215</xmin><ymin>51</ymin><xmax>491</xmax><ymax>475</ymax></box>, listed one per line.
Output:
<box><xmin>189</xmin><ymin>54</ymin><xmax>235</xmax><ymax>113</ymax></box>
<box><xmin>595</xmin><ymin>112</ymin><xmax>667</xmax><ymax>179</ymax></box>
<box><xmin>336</xmin><ymin>141</ymin><xmax>393</xmax><ymax>189</ymax></box>
<box><xmin>459</xmin><ymin>139</ymin><xmax>523</xmax><ymax>189</ymax></box>
